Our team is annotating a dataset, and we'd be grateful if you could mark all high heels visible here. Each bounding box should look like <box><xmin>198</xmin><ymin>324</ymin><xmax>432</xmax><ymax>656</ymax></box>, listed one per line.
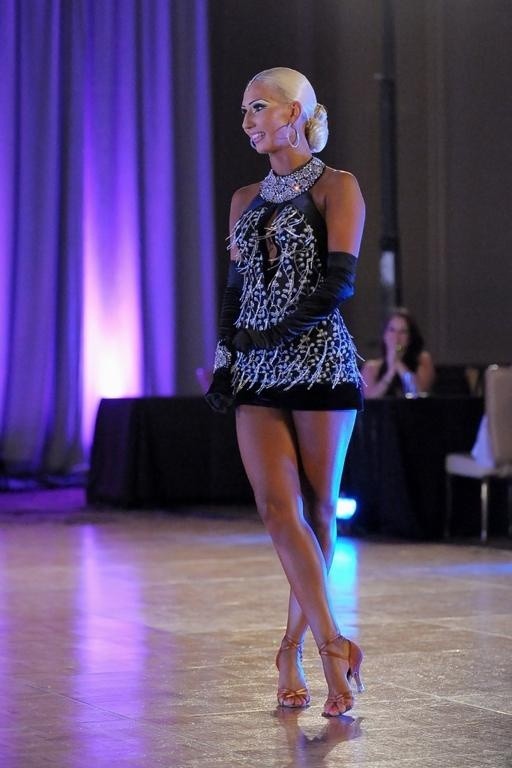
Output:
<box><xmin>318</xmin><ymin>634</ymin><xmax>364</xmax><ymax>718</ymax></box>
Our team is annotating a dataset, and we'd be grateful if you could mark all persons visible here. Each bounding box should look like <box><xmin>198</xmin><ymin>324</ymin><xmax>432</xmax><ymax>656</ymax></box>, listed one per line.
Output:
<box><xmin>356</xmin><ymin>299</ymin><xmax>440</xmax><ymax>400</ymax></box>
<box><xmin>207</xmin><ymin>67</ymin><xmax>366</xmax><ymax>721</ymax></box>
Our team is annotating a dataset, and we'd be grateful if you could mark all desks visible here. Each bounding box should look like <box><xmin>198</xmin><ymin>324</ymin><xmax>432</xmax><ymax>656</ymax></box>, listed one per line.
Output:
<box><xmin>85</xmin><ymin>394</ymin><xmax>480</xmax><ymax>540</ymax></box>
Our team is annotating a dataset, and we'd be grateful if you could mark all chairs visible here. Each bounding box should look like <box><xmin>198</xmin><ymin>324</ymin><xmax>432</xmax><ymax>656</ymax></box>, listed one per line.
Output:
<box><xmin>433</xmin><ymin>364</ymin><xmax>512</xmax><ymax>543</ymax></box>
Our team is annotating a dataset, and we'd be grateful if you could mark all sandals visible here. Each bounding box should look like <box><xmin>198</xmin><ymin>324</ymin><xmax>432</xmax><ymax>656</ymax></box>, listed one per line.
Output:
<box><xmin>275</xmin><ymin>634</ymin><xmax>311</xmax><ymax>708</ymax></box>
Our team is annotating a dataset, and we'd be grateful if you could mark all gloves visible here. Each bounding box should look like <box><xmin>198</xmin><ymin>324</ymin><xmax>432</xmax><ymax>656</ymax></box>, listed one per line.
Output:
<box><xmin>228</xmin><ymin>250</ymin><xmax>358</xmax><ymax>355</ymax></box>
<box><xmin>204</xmin><ymin>259</ymin><xmax>246</xmax><ymax>413</ymax></box>
